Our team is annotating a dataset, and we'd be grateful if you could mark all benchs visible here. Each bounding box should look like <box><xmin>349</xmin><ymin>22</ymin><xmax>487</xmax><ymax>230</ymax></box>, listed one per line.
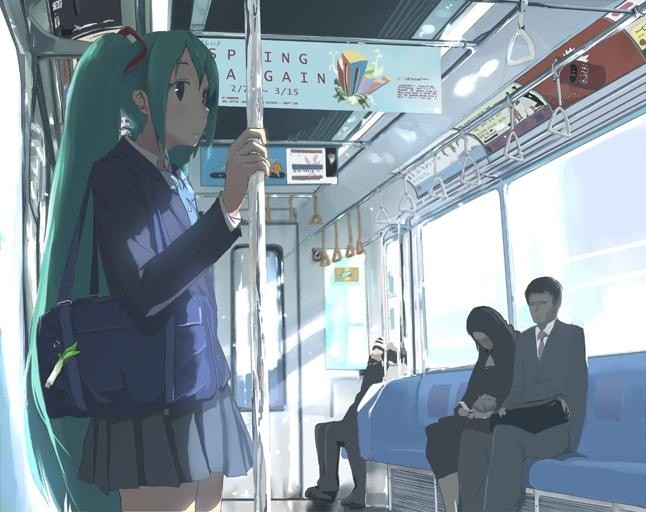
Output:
<box><xmin>354</xmin><ymin>350</ymin><xmax>645</xmax><ymax>510</ymax></box>
